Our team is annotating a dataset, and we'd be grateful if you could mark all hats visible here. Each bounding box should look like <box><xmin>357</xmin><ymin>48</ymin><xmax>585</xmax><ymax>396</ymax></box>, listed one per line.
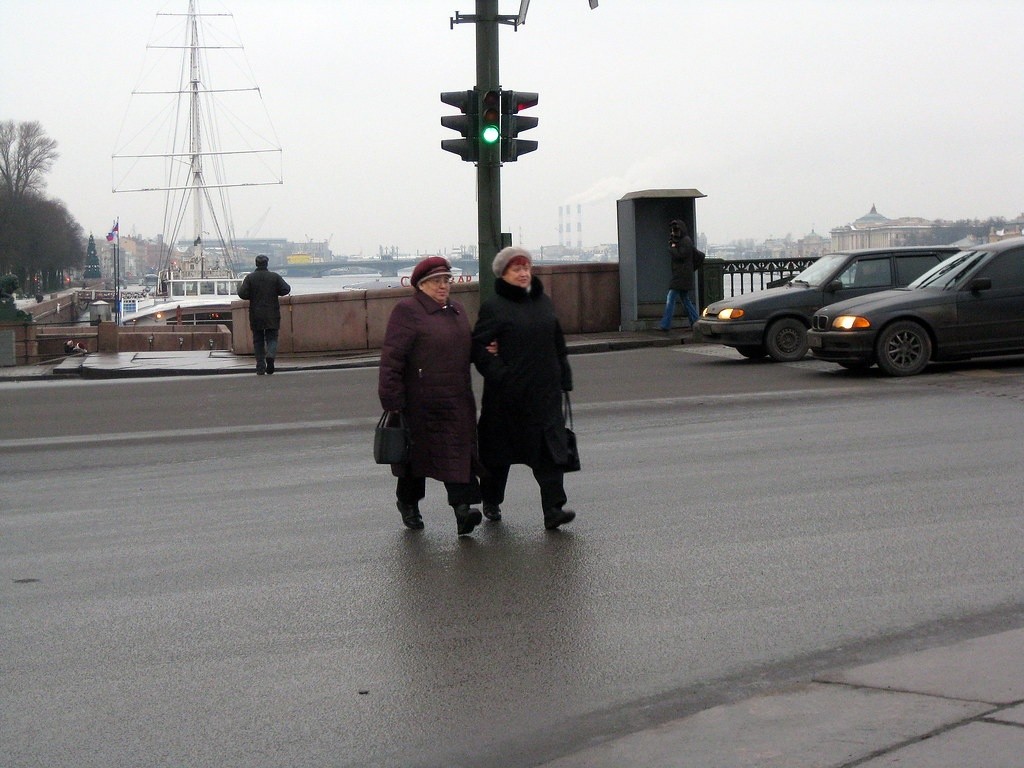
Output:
<box><xmin>255</xmin><ymin>254</ymin><xmax>269</xmax><ymax>265</ymax></box>
<box><xmin>492</xmin><ymin>247</ymin><xmax>532</xmax><ymax>278</ymax></box>
<box><xmin>411</xmin><ymin>257</ymin><xmax>452</xmax><ymax>289</ymax></box>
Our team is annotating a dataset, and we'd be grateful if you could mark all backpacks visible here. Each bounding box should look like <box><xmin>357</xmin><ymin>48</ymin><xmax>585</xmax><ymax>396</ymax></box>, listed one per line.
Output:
<box><xmin>678</xmin><ymin>242</ymin><xmax>705</xmax><ymax>271</ymax></box>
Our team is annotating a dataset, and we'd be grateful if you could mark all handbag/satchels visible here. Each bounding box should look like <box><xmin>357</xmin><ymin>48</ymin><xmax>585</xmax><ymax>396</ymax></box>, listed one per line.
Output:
<box><xmin>563</xmin><ymin>391</ymin><xmax>581</xmax><ymax>472</ymax></box>
<box><xmin>374</xmin><ymin>410</ymin><xmax>413</xmax><ymax>463</ymax></box>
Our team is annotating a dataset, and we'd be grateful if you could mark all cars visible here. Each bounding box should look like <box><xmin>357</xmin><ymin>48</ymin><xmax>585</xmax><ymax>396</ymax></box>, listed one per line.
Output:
<box><xmin>807</xmin><ymin>237</ymin><xmax>1024</xmax><ymax>376</ymax></box>
<box><xmin>692</xmin><ymin>244</ymin><xmax>961</xmax><ymax>362</ymax></box>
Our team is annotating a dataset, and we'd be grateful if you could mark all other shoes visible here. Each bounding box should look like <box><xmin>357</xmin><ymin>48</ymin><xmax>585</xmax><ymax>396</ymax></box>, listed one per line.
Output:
<box><xmin>257</xmin><ymin>368</ymin><xmax>265</xmax><ymax>375</ymax></box>
<box><xmin>652</xmin><ymin>326</ymin><xmax>669</xmax><ymax>332</ymax></box>
<box><xmin>544</xmin><ymin>506</ymin><xmax>576</xmax><ymax>529</ymax></box>
<box><xmin>396</xmin><ymin>500</ymin><xmax>424</xmax><ymax>529</ymax></box>
<box><xmin>483</xmin><ymin>501</ymin><xmax>501</xmax><ymax>519</ymax></box>
<box><xmin>266</xmin><ymin>357</ymin><xmax>275</xmax><ymax>374</ymax></box>
<box><xmin>454</xmin><ymin>503</ymin><xmax>482</xmax><ymax>534</ymax></box>
<box><xmin>685</xmin><ymin>328</ymin><xmax>693</xmax><ymax>331</ymax></box>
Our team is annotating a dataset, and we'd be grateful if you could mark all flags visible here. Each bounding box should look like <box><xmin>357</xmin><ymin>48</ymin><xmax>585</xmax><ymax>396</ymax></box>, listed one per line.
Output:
<box><xmin>106</xmin><ymin>219</ymin><xmax>119</xmax><ymax>245</ymax></box>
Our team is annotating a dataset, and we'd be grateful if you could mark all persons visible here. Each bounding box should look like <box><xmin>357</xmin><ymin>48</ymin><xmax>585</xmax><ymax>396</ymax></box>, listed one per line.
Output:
<box><xmin>238</xmin><ymin>255</ymin><xmax>291</xmax><ymax>377</ymax></box>
<box><xmin>471</xmin><ymin>248</ymin><xmax>582</xmax><ymax>531</ymax></box>
<box><xmin>650</xmin><ymin>219</ymin><xmax>700</xmax><ymax>336</ymax></box>
<box><xmin>373</xmin><ymin>256</ymin><xmax>484</xmax><ymax>535</ymax></box>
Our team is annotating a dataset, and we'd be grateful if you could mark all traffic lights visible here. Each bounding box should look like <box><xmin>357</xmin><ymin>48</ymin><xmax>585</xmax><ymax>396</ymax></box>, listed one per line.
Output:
<box><xmin>508</xmin><ymin>90</ymin><xmax>539</xmax><ymax>162</ymax></box>
<box><xmin>440</xmin><ymin>90</ymin><xmax>477</xmax><ymax>162</ymax></box>
<box><xmin>480</xmin><ymin>89</ymin><xmax>500</xmax><ymax>144</ymax></box>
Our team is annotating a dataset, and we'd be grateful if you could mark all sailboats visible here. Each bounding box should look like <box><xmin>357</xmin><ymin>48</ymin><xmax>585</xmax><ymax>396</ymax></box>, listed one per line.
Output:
<box><xmin>110</xmin><ymin>0</ymin><xmax>287</xmax><ymax>326</ymax></box>
